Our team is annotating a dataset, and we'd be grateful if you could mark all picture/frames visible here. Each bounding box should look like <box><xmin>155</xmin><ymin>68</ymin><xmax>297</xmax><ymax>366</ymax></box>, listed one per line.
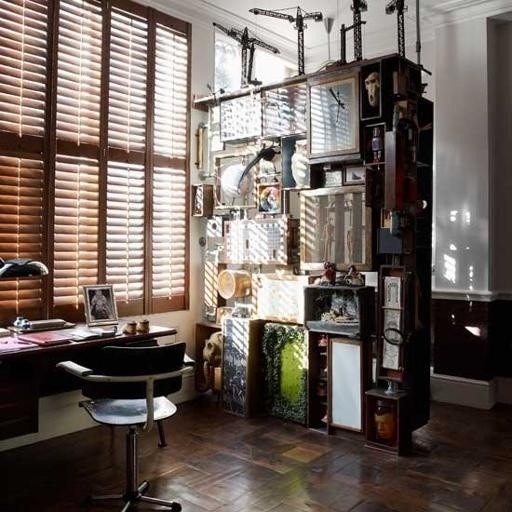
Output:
<box><xmin>260</xmin><ymin>82</ymin><xmax>307</xmax><ymax>139</ymax></box>
<box><xmin>299</xmin><ymin>185</ymin><xmax>373</xmax><ymax>271</ymax></box>
<box><xmin>306</xmin><ymin>70</ymin><xmax>360</xmax><ymax>159</ymax></box>
<box><xmin>223</xmin><ymin>216</ymin><xmax>288</xmax><ymax>264</ymax></box>
<box><xmin>218</xmin><ymin>90</ymin><xmax>261</xmax><ymax>143</ymax></box>
<box><xmin>256</xmin><ymin>181</ymin><xmax>282</xmax><ymax>215</ymax></box>
<box><xmin>210</xmin><ymin>150</ymin><xmax>258</xmax><ymax>211</ymax></box>
<box><xmin>341</xmin><ymin>165</ymin><xmax>366</xmax><ymax>186</ymax></box>
<box><xmin>83</xmin><ymin>285</ymin><xmax>118</xmax><ymax>327</ymax></box>
<box><xmin>250</xmin><ymin>273</ymin><xmax>305</xmax><ymax>325</ymax></box>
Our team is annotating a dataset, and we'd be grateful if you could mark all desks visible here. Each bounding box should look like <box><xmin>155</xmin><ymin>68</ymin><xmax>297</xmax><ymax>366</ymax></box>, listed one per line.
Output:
<box><xmin>0</xmin><ymin>319</ymin><xmax>178</xmax><ymax>448</ymax></box>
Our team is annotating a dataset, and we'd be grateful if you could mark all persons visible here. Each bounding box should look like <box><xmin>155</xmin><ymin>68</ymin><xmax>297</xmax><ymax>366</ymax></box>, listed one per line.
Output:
<box><xmin>91</xmin><ymin>289</ymin><xmax>110</xmax><ymax>319</ymax></box>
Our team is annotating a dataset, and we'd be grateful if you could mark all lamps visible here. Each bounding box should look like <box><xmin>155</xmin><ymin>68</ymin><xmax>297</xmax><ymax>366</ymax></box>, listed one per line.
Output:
<box><xmin>0</xmin><ymin>258</ymin><xmax>49</xmax><ymax>277</ymax></box>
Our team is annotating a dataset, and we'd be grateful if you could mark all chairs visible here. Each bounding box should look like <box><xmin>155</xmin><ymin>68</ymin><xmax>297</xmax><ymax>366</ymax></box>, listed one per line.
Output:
<box><xmin>55</xmin><ymin>341</ymin><xmax>196</xmax><ymax>512</ymax></box>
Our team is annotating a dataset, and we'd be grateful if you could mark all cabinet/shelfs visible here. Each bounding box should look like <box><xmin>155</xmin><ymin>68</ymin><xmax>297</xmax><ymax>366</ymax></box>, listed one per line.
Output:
<box><xmin>191</xmin><ymin>53</ymin><xmax>434</xmax><ymax>458</ymax></box>
